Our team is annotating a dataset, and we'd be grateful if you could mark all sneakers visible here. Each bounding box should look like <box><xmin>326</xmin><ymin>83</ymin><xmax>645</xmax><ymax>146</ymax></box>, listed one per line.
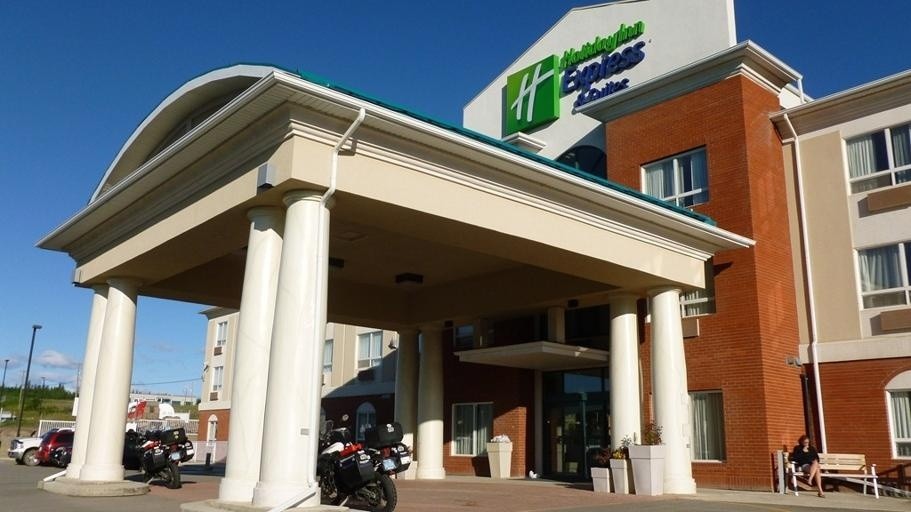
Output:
<box><xmin>807</xmin><ymin>481</ymin><xmax>826</xmax><ymax>498</ymax></box>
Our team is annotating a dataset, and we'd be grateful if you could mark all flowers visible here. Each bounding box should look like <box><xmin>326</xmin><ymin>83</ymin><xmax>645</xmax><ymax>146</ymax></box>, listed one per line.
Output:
<box><xmin>594</xmin><ymin>420</ymin><xmax>666</xmax><ymax>467</ymax></box>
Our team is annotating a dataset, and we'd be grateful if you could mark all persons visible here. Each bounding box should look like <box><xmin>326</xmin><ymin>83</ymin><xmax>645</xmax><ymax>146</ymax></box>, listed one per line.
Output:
<box><xmin>791</xmin><ymin>435</ymin><xmax>826</xmax><ymax>498</ymax></box>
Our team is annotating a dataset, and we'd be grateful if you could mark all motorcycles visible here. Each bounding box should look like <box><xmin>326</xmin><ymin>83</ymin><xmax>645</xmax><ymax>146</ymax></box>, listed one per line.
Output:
<box><xmin>129</xmin><ymin>428</ymin><xmax>194</xmax><ymax>491</ymax></box>
<box><xmin>313</xmin><ymin>409</ymin><xmax>414</xmax><ymax>511</ymax></box>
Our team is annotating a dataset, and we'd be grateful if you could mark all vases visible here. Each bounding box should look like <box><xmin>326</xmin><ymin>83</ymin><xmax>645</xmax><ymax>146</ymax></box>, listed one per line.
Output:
<box><xmin>591</xmin><ymin>444</ymin><xmax>666</xmax><ymax>497</ymax></box>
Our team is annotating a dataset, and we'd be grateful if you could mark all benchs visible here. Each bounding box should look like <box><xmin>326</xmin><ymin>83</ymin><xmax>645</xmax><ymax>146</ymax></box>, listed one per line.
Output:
<box><xmin>783</xmin><ymin>451</ymin><xmax>881</xmax><ymax>498</ymax></box>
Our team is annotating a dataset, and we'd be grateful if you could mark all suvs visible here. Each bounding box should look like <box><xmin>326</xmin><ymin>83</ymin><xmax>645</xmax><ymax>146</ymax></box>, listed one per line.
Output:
<box><xmin>5</xmin><ymin>425</ymin><xmax>142</xmax><ymax>472</ymax></box>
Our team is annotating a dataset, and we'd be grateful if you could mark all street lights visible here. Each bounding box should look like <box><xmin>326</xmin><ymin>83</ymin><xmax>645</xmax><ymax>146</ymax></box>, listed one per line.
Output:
<box><xmin>1</xmin><ymin>322</ymin><xmax>46</xmax><ymax>437</ymax></box>
